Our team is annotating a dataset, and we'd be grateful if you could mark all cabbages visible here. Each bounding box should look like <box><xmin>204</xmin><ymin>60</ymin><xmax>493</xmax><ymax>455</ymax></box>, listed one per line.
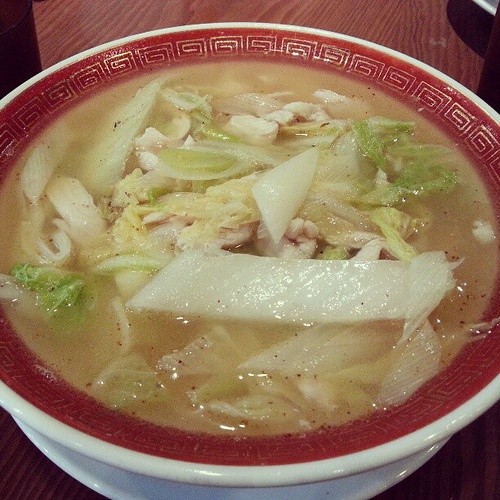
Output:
<box><xmin>11</xmin><ymin>77</ymin><xmax>461</xmax><ymax>416</ymax></box>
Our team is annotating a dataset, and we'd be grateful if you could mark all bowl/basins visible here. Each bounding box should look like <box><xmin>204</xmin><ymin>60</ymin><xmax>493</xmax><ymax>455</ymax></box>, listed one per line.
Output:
<box><xmin>0</xmin><ymin>21</ymin><xmax>498</xmax><ymax>498</ymax></box>
